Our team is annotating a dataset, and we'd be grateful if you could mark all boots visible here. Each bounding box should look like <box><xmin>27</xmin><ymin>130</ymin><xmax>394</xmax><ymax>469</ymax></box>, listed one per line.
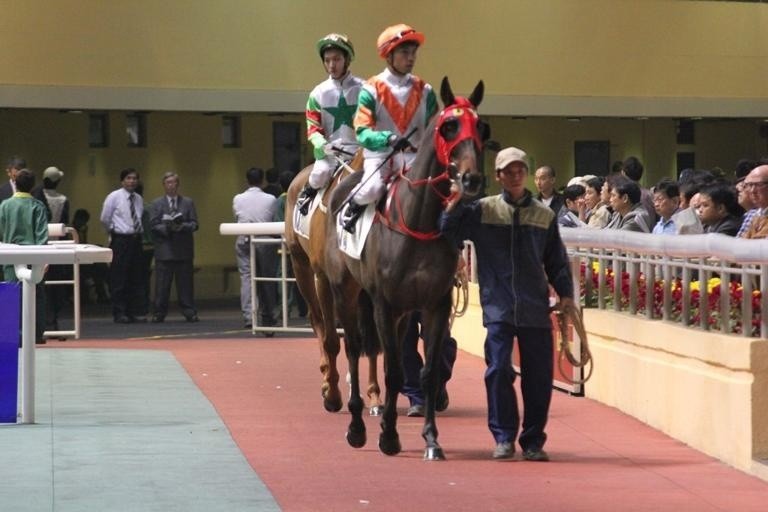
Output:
<box><xmin>340</xmin><ymin>192</ymin><xmax>368</xmax><ymax>235</ymax></box>
<box><xmin>296</xmin><ymin>179</ymin><xmax>321</xmax><ymax>218</ymax></box>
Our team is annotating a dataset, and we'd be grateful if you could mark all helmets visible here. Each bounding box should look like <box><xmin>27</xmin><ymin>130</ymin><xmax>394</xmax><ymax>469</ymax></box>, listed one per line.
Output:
<box><xmin>376</xmin><ymin>23</ymin><xmax>426</xmax><ymax>60</ymax></box>
<box><xmin>314</xmin><ymin>33</ymin><xmax>356</xmax><ymax>63</ymax></box>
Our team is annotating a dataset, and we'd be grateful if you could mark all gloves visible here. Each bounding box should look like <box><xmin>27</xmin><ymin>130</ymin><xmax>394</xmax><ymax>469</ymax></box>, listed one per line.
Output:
<box><xmin>323</xmin><ymin>138</ymin><xmax>344</xmax><ymax>154</ymax></box>
<box><xmin>386</xmin><ymin>133</ymin><xmax>411</xmax><ymax>153</ymax></box>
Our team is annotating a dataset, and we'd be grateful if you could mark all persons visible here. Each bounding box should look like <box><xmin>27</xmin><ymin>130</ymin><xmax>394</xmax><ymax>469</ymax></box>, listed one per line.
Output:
<box><xmin>2</xmin><ymin>169</ymin><xmax>49</xmax><ymax>341</ymax></box>
<box><xmin>1</xmin><ymin>156</ymin><xmax>59</xmax><ymax>339</ymax></box>
<box><xmin>296</xmin><ymin>33</ymin><xmax>367</xmax><ymax>216</ymax></box>
<box><xmin>101</xmin><ymin>168</ymin><xmax>150</xmax><ymax>324</ymax></box>
<box><xmin>341</xmin><ymin>22</ymin><xmax>439</xmax><ymax>234</ymax></box>
<box><xmin>42</xmin><ymin>167</ymin><xmax>69</xmax><ymax>241</ymax></box>
<box><xmin>534</xmin><ymin>156</ymin><xmax>767</xmax><ymax>289</ymax></box>
<box><xmin>234</xmin><ymin>167</ymin><xmax>307</xmax><ymax>337</ymax></box>
<box><xmin>451</xmin><ymin>147</ymin><xmax>573</xmax><ymax>460</ymax></box>
<box><xmin>149</xmin><ymin>172</ymin><xmax>199</xmax><ymax>321</ymax></box>
<box><xmin>67</xmin><ymin>208</ymin><xmax>111</xmax><ymax>305</ymax></box>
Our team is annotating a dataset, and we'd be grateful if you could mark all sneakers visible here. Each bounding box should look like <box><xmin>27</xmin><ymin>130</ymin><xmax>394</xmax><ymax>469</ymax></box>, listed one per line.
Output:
<box><xmin>434</xmin><ymin>386</ymin><xmax>450</xmax><ymax>412</ymax></box>
<box><xmin>406</xmin><ymin>400</ymin><xmax>428</xmax><ymax>417</ymax></box>
<box><xmin>492</xmin><ymin>440</ymin><xmax>515</xmax><ymax>461</ymax></box>
<box><xmin>244</xmin><ymin>319</ymin><xmax>254</xmax><ymax>328</ymax></box>
<box><xmin>521</xmin><ymin>445</ymin><xmax>549</xmax><ymax>462</ymax></box>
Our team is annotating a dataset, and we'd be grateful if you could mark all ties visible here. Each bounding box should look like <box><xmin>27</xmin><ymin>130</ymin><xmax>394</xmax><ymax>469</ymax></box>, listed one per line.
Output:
<box><xmin>169</xmin><ymin>198</ymin><xmax>177</xmax><ymax>215</ymax></box>
<box><xmin>128</xmin><ymin>193</ymin><xmax>141</xmax><ymax>234</ymax></box>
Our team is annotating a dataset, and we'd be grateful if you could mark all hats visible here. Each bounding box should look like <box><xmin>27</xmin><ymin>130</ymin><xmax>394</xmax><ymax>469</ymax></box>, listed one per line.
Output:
<box><xmin>42</xmin><ymin>166</ymin><xmax>65</xmax><ymax>183</ymax></box>
<box><xmin>567</xmin><ymin>174</ymin><xmax>598</xmax><ymax>191</ymax></box>
<box><xmin>494</xmin><ymin>146</ymin><xmax>530</xmax><ymax>172</ymax></box>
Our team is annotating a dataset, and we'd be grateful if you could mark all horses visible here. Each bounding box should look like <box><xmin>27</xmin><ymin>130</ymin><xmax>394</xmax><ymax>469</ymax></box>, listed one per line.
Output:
<box><xmin>284</xmin><ymin>146</ymin><xmax>385</xmax><ymax>416</ymax></box>
<box><xmin>322</xmin><ymin>75</ymin><xmax>491</xmax><ymax>462</ymax></box>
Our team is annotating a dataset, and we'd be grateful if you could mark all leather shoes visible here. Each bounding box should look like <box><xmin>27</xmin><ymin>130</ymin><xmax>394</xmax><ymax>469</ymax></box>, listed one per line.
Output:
<box><xmin>152</xmin><ymin>313</ymin><xmax>167</xmax><ymax>324</ymax></box>
<box><xmin>185</xmin><ymin>311</ymin><xmax>200</xmax><ymax>324</ymax></box>
<box><xmin>113</xmin><ymin>312</ymin><xmax>148</xmax><ymax>324</ymax></box>
